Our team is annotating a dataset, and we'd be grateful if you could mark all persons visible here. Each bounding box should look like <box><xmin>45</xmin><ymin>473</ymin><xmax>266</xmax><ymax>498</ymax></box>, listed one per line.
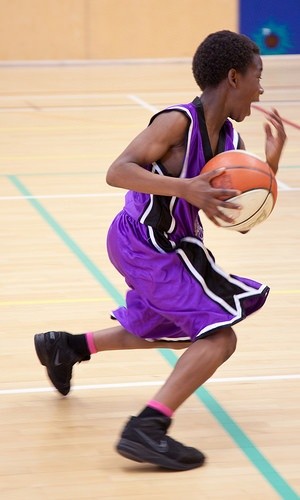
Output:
<box><xmin>35</xmin><ymin>30</ymin><xmax>286</xmax><ymax>472</ymax></box>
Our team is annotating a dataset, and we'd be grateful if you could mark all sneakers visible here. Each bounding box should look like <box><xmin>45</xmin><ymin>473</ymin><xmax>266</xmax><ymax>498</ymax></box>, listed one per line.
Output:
<box><xmin>114</xmin><ymin>415</ymin><xmax>204</xmax><ymax>468</ymax></box>
<box><xmin>33</xmin><ymin>330</ymin><xmax>74</xmax><ymax>396</ymax></box>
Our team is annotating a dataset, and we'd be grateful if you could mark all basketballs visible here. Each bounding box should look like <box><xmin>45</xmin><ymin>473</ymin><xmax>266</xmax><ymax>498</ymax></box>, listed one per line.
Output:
<box><xmin>199</xmin><ymin>149</ymin><xmax>278</xmax><ymax>232</ymax></box>
